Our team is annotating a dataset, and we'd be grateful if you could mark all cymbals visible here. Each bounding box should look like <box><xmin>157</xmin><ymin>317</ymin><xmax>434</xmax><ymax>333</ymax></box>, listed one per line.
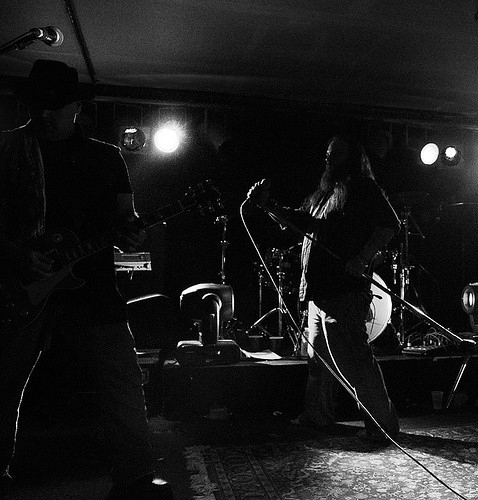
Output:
<box><xmin>400</xmin><ymin>230</ymin><xmax>425</xmax><ymax>236</ymax></box>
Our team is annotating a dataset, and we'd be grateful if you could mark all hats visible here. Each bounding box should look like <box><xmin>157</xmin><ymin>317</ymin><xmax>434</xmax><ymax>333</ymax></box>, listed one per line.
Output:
<box><xmin>14</xmin><ymin>59</ymin><xmax>96</xmax><ymax>110</ymax></box>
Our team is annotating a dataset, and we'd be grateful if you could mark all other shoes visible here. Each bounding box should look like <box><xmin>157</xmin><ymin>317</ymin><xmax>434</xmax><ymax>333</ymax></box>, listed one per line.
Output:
<box><xmin>314</xmin><ymin>424</ymin><xmax>334</xmax><ymax>434</ymax></box>
<box><xmin>368</xmin><ymin>434</ymin><xmax>397</xmax><ymax>449</ymax></box>
<box><xmin>107</xmin><ymin>477</ymin><xmax>172</xmax><ymax>500</ymax></box>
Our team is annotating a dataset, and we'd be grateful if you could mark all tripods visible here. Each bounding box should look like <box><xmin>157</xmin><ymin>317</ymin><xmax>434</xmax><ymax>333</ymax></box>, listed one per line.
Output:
<box><xmin>385</xmin><ymin>216</ymin><xmax>435</xmax><ymax>344</ymax></box>
<box><xmin>215</xmin><ymin>217</ymin><xmax>305</xmax><ymax>357</ymax></box>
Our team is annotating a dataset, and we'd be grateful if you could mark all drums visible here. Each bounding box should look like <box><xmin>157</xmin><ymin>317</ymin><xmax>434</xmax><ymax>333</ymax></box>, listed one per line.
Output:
<box><xmin>297</xmin><ymin>270</ymin><xmax>393</xmax><ymax>344</ymax></box>
<box><xmin>374</xmin><ymin>250</ymin><xmax>410</xmax><ymax>287</ymax></box>
<box><xmin>260</xmin><ymin>247</ymin><xmax>301</xmax><ymax>293</ymax></box>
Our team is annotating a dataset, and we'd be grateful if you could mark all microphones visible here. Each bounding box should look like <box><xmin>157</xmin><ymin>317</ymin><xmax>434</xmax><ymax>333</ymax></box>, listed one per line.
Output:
<box><xmin>32</xmin><ymin>26</ymin><xmax>64</xmax><ymax>46</ymax></box>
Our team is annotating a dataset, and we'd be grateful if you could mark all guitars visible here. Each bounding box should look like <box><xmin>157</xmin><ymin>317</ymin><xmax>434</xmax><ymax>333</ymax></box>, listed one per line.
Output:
<box><xmin>0</xmin><ymin>179</ymin><xmax>224</xmax><ymax>334</ymax></box>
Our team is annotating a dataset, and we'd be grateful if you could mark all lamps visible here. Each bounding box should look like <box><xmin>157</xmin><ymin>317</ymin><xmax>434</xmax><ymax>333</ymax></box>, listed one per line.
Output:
<box><xmin>112</xmin><ymin>103</ymin><xmax>146</xmax><ymax>151</ymax></box>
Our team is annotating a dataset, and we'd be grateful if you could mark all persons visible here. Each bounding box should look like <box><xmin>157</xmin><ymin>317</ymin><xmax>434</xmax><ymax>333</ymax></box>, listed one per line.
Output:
<box><xmin>0</xmin><ymin>59</ymin><xmax>174</xmax><ymax>500</ymax></box>
<box><xmin>246</xmin><ymin>133</ymin><xmax>406</xmax><ymax>445</ymax></box>
